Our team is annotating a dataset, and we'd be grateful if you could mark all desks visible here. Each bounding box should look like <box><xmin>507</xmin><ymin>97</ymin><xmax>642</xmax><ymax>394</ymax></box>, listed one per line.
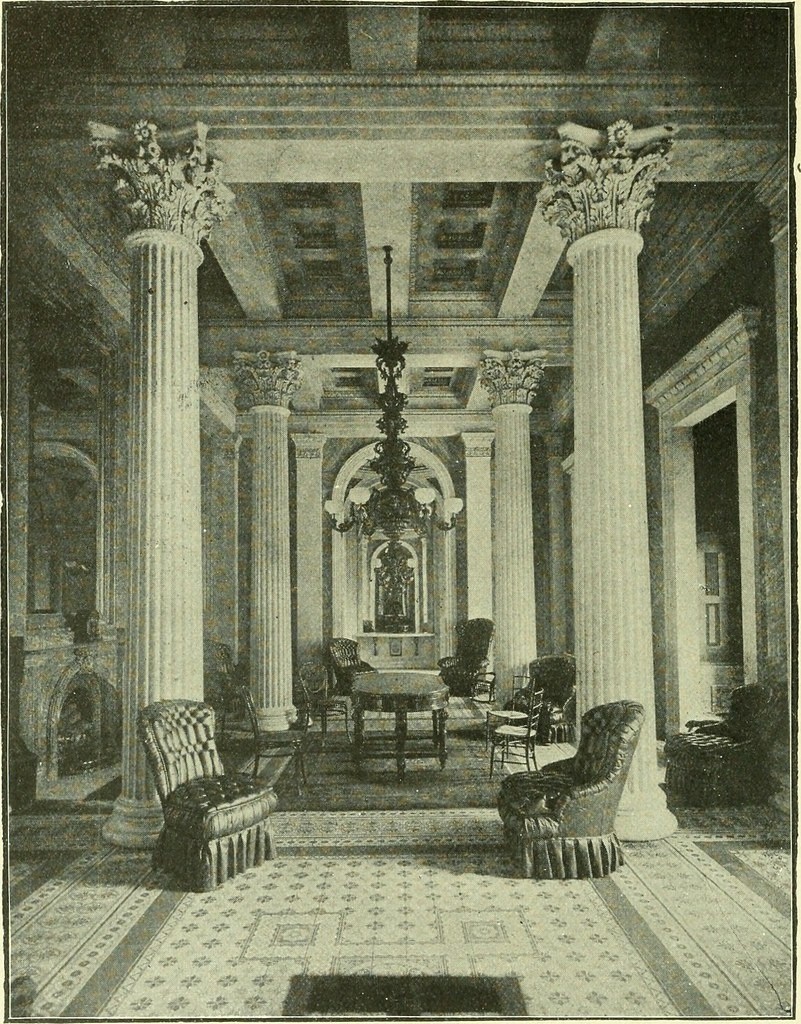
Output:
<box><xmin>350</xmin><ymin>671</ymin><xmax>451</xmax><ymax>784</ymax></box>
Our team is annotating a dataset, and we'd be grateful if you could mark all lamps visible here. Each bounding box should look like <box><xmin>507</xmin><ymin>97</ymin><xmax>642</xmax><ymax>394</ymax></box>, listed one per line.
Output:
<box><xmin>312</xmin><ymin>244</ymin><xmax>464</xmax><ymax>543</ymax></box>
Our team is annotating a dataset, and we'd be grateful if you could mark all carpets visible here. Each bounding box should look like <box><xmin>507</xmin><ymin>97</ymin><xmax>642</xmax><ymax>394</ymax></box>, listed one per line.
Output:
<box><xmin>265</xmin><ymin>732</ymin><xmax>514</xmax><ymax>813</ymax></box>
<box><xmin>282</xmin><ymin>973</ymin><xmax>527</xmax><ymax>1016</ymax></box>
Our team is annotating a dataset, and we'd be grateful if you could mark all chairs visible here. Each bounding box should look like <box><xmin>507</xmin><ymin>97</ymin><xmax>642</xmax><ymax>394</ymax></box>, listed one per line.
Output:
<box><xmin>299</xmin><ymin>661</ymin><xmax>355</xmax><ymax>749</ymax></box>
<box><xmin>239</xmin><ymin>685</ymin><xmax>309</xmax><ymax>788</ymax></box>
<box><xmin>485</xmin><ymin>675</ymin><xmax>537</xmax><ymax>755</ymax></box>
<box><xmin>470</xmin><ymin>669</ymin><xmax>496</xmax><ymax>710</ymax></box>
<box><xmin>488</xmin><ymin>686</ymin><xmax>545</xmax><ymax>780</ymax></box>
<box><xmin>216</xmin><ymin>660</ymin><xmax>262</xmax><ymax>740</ymax></box>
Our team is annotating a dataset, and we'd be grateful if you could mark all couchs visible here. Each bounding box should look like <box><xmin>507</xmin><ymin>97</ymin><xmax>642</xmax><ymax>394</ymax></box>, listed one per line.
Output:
<box><xmin>327</xmin><ymin>638</ymin><xmax>379</xmax><ymax>697</ymax></box>
<box><xmin>508</xmin><ymin>652</ymin><xmax>576</xmax><ymax>744</ymax></box>
<box><xmin>496</xmin><ymin>700</ymin><xmax>646</xmax><ymax>881</ymax></box>
<box><xmin>136</xmin><ymin>699</ymin><xmax>279</xmax><ymax>893</ymax></box>
<box><xmin>663</xmin><ymin>681</ymin><xmax>778</xmax><ymax>809</ymax></box>
<box><xmin>436</xmin><ymin>618</ymin><xmax>494</xmax><ymax>698</ymax></box>
